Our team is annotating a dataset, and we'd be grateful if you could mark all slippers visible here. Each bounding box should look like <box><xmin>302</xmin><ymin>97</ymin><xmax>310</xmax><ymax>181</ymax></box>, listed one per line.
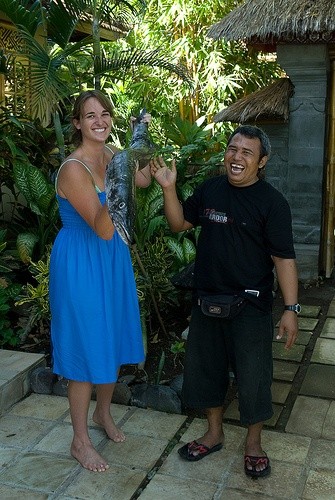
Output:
<box><xmin>244</xmin><ymin>451</ymin><xmax>271</xmax><ymax>477</ymax></box>
<box><xmin>178</xmin><ymin>438</ymin><xmax>222</xmax><ymax>461</ymax></box>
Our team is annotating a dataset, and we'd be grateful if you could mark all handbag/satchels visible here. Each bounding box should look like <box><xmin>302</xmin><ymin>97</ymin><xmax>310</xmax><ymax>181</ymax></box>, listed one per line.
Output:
<box><xmin>200</xmin><ymin>294</ymin><xmax>245</xmax><ymax>318</ymax></box>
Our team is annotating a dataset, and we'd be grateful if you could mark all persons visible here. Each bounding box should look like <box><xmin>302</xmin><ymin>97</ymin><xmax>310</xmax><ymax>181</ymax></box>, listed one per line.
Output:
<box><xmin>47</xmin><ymin>89</ymin><xmax>153</xmax><ymax>471</ymax></box>
<box><xmin>149</xmin><ymin>125</ymin><xmax>303</xmax><ymax>477</ymax></box>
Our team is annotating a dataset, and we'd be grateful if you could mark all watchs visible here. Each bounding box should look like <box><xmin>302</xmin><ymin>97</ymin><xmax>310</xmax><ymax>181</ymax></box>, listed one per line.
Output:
<box><xmin>283</xmin><ymin>303</ymin><xmax>302</xmax><ymax>314</ymax></box>
